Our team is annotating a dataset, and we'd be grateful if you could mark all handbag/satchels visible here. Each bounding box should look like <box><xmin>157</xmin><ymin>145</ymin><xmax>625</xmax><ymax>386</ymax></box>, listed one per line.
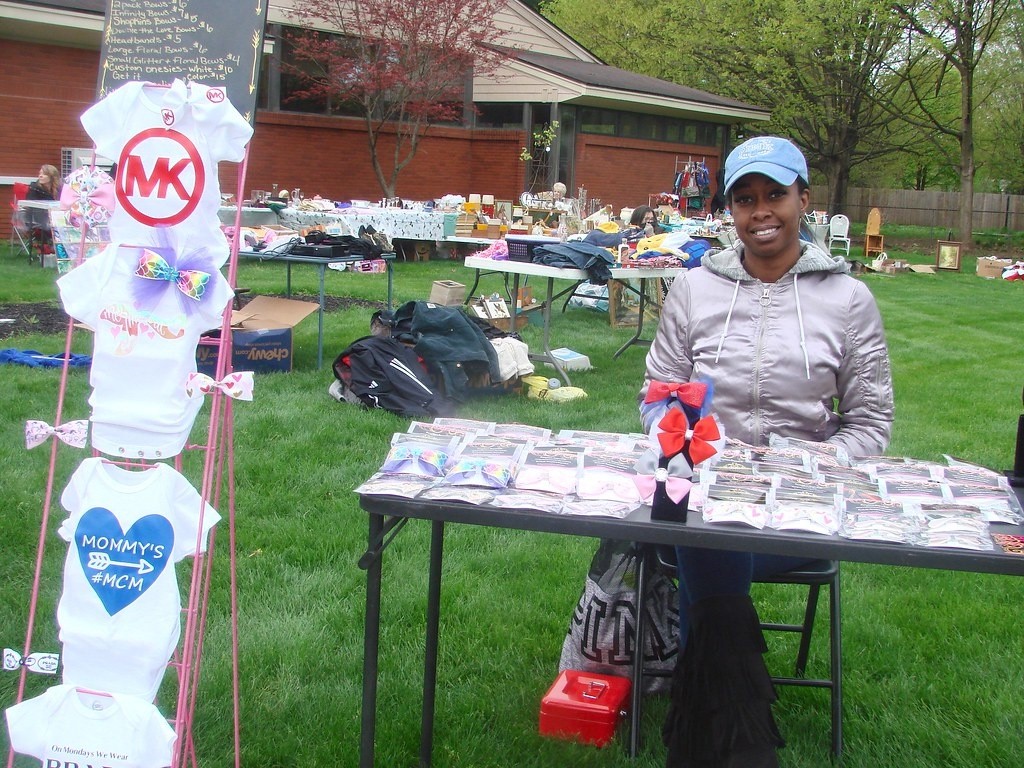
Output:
<box><xmin>558</xmin><ymin>537</ymin><xmax>680</xmax><ymax>705</ymax></box>
<box><xmin>682</xmin><ymin>168</ymin><xmax>711</xmax><ymax>209</ymax></box>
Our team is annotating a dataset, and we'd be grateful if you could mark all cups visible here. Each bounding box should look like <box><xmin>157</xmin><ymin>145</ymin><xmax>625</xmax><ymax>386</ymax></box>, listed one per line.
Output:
<box><xmin>378</xmin><ymin>197</ymin><xmax>438</xmax><ymax>210</ymax></box>
<box><xmin>251</xmin><ymin>190</ymin><xmax>264</xmax><ymax>206</ymax></box>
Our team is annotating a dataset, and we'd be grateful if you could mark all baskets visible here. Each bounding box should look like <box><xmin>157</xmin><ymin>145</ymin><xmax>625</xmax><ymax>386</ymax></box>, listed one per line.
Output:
<box><xmin>505</xmin><ymin>237</ymin><xmax>561</xmax><ymax>264</ymax></box>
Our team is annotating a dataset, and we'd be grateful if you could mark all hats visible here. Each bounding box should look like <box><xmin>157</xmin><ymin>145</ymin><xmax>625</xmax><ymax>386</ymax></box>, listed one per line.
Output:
<box><xmin>723</xmin><ymin>136</ymin><xmax>809</xmax><ymax>197</ymax></box>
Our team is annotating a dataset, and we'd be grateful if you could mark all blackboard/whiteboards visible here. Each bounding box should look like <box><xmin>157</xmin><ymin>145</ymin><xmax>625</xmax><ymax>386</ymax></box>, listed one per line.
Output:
<box><xmin>96</xmin><ymin>0</ymin><xmax>270</xmax><ymax>131</ymax></box>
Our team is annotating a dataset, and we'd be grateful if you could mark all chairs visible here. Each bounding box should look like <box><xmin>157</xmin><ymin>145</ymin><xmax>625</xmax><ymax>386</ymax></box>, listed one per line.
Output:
<box><xmin>628</xmin><ymin>542</ymin><xmax>843</xmax><ymax>761</ymax></box>
<box><xmin>7</xmin><ymin>181</ymin><xmax>52</xmax><ymax>263</ymax></box>
<box><xmin>828</xmin><ymin>215</ymin><xmax>850</xmax><ymax>256</ymax></box>
<box><xmin>863</xmin><ymin>208</ymin><xmax>884</xmax><ymax>258</ymax></box>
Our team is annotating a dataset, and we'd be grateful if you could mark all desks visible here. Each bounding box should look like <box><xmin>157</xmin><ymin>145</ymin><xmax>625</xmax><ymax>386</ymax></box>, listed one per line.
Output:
<box><xmin>463</xmin><ymin>254</ymin><xmax>609</xmax><ymax>387</ymax></box>
<box><xmin>278</xmin><ymin>209</ymin><xmax>497</xmax><ymax>244</ymax></box>
<box><xmin>219</xmin><ymin>206</ymin><xmax>278</xmax><ymax>227</ymax></box>
<box><xmin>239</xmin><ymin>252</ymin><xmax>396</xmax><ymax>368</ymax></box>
<box><xmin>608</xmin><ymin>268</ymin><xmax>689</xmax><ymax>359</ymax></box>
<box><xmin>357</xmin><ymin>418</ymin><xmax>1024</xmax><ymax>768</ymax></box>
<box><xmin>17</xmin><ymin>199</ymin><xmax>60</xmax><ymax>268</ymax></box>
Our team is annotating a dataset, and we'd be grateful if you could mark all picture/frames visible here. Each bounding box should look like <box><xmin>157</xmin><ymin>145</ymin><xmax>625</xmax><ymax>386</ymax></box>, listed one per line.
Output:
<box><xmin>936</xmin><ymin>240</ymin><xmax>963</xmax><ymax>273</ymax></box>
<box><xmin>494</xmin><ymin>199</ymin><xmax>513</xmax><ymax>220</ymax></box>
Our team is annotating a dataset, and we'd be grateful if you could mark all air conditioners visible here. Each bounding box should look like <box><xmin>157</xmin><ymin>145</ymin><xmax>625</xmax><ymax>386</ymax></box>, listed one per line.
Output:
<box><xmin>61</xmin><ymin>147</ymin><xmax>92</xmax><ymax>178</ymax></box>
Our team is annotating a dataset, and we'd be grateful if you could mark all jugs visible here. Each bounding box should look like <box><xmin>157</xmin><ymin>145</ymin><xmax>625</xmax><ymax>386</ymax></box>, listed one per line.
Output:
<box><xmin>291</xmin><ymin>188</ymin><xmax>301</xmax><ymax>206</ymax></box>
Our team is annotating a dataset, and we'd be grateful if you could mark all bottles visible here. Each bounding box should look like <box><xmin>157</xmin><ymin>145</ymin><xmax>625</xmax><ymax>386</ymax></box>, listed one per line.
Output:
<box><xmin>618</xmin><ymin>238</ymin><xmax>629</xmax><ymax>263</ymax></box>
<box><xmin>271</xmin><ymin>183</ymin><xmax>278</xmax><ymax>197</ymax></box>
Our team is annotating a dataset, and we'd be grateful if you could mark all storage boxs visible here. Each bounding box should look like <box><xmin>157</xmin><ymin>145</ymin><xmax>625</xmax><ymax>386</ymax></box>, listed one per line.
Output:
<box><xmin>505</xmin><ymin>234</ymin><xmax>561</xmax><ymax>263</ymax></box>
<box><xmin>429</xmin><ymin>280</ymin><xmax>466</xmax><ymax>308</ymax></box>
<box><xmin>196</xmin><ymin>293</ymin><xmax>321</xmax><ymax>375</ymax></box>
<box><xmin>543</xmin><ymin>347</ymin><xmax>593</xmax><ymax>371</ymax></box>
<box><xmin>976</xmin><ymin>259</ymin><xmax>1010</xmax><ymax>278</ymax></box>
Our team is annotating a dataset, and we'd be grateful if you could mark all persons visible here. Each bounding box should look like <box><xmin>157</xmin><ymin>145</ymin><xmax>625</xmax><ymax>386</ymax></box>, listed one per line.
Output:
<box><xmin>636</xmin><ymin>137</ymin><xmax>894</xmax><ymax>768</ymax></box>
<box><xmin>25</xmin><ymin>164</ymin><xmax>61</xmax><ymax>226</ymax></box>
<box><xmin>631</xmin><ymin>204</ymin><xmax>658</xmax><ymax>237</ymax></box>
<box><xmin>554</xmin><ymin>182</ymin><xmax>566</xmax><ymax>201</ymax></box>
